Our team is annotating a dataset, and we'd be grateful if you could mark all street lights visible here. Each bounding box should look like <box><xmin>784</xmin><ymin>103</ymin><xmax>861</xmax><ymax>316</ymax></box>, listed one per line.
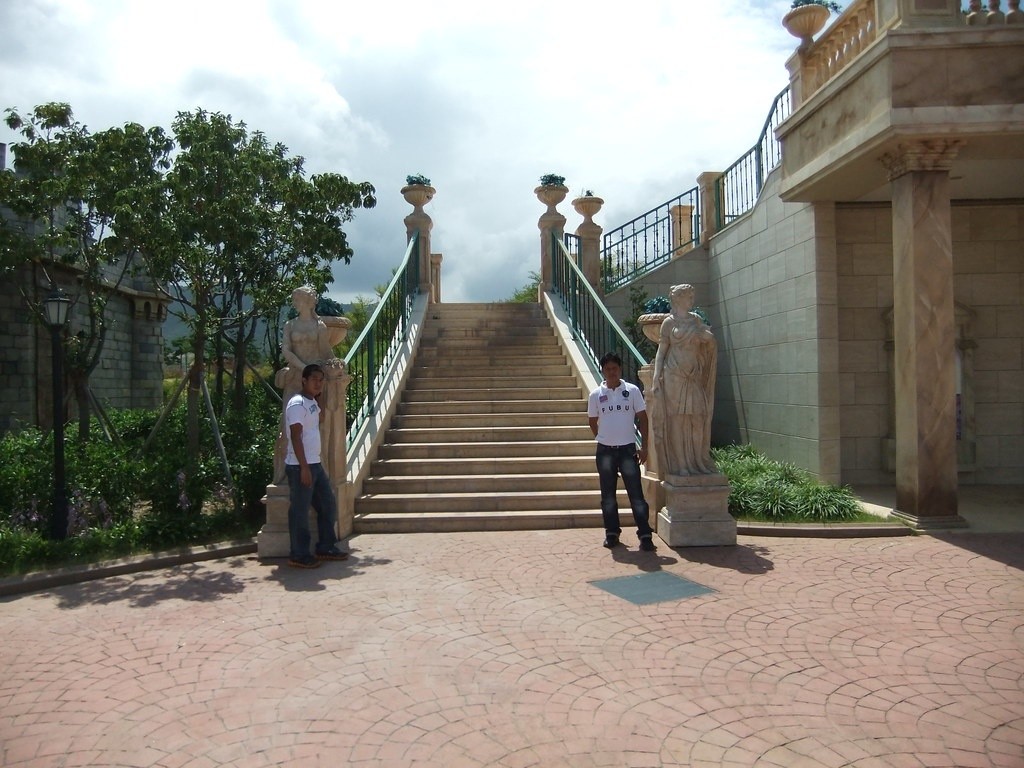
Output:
<box><xmin>42</xmin><ymin>281</ymin><xmax>71</xmax><ymax>538</ymax></box>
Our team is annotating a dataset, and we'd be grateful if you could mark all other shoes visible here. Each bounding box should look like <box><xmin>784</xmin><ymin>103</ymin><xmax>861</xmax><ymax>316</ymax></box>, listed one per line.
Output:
<box><xmin>639</xmin><ymin>539</ymin><xmax>657</xmax><ymax>550</ymax></box>
<box><xmin>603</xmin><ymin>536</ymin><xmax>619</xmax><ymax>548</ymax></box>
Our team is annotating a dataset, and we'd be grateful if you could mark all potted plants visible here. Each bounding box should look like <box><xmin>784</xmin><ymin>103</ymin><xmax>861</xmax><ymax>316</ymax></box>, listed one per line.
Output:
<box><xmin>636</xmin><ymin>296</ymin><xmax>710</xmax><ymax>370</ymax></box>
<box><xmin>533</xmin><ymin>174</ymin><xmax>568</xmax><ymax>220</ymax></box>
<box><xmin>400</xmin><ymin>174</ymin><xmax>436</xmax><ymax>220</ymax></box>
<box><xmin>571</xmin><ymin>190</ymin><xmax>604</xmax><ymax>228</ymax></box>
<box><xmin>282</xmin><ymin>298</ymin><xmax>350</xmax><ymax>349</ymax></box>
<box><xmin>782</xmin><ymin>0</ymin><xmax>843</xmax><ymax>51</ymax></box>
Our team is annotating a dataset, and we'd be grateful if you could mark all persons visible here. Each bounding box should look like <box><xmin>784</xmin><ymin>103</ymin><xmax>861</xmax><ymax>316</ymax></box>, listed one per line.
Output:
<box><xmin>653</xmin><ymin>284</ymin><xmax>717</xmax><ymax>473</ymax></box>
<box><xmin>283</xmin><ymin>365</ymin><xmax>349</xmax><ymax>567</ymax></box>
<box><xmin>274</xmin><ymin>287</ymin><xmax>347</xmax><ymax>481</ymax></box>
<box><xmin>587</xmin><ymin>354</ymin><xmax>657</xmax><ymax>551</ymax></box>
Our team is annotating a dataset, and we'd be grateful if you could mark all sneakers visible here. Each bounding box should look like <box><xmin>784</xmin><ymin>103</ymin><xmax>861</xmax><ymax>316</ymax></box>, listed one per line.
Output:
<box><xmin>316</xmin><ymin>547</ymin><xmax>350</xmax><ymax>560</ymax></box>
<box><xmin>287</xmin><ymin>553</ymin><xmax>322</xmax><ymax>568</ymax></box>
